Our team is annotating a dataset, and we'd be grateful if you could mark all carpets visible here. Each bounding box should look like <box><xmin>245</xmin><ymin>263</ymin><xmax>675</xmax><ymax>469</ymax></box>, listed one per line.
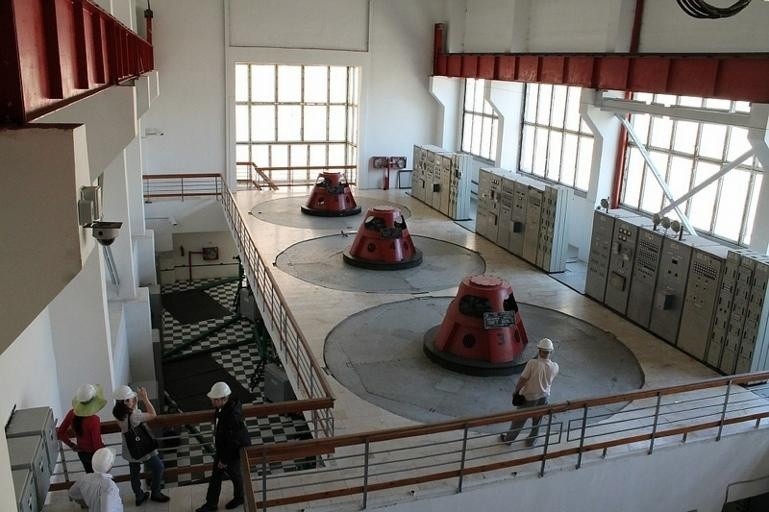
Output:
<box><xmin>153</xmin><ymin>349</ymin><xmax>257</xmax><ymax>414</ymax></box>
<box><xmin>162</xmin><ymin>285</ymin><xmax>233</xmax><ymax>325</ymax></box>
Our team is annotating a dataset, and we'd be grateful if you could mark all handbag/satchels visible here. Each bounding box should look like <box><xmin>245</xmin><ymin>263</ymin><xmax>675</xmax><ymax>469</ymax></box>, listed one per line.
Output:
<box><xmin>123</xmin><ymin>412</ymin><xmax>159</xmax><ymax>459</ymax></box>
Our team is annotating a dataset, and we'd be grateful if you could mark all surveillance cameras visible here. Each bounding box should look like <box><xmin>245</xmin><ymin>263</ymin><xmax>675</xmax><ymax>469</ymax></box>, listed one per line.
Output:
<box><xmin>93</xmin><ymin>222</ymin><xmax>123</xmax><ymax>246</ymax></box>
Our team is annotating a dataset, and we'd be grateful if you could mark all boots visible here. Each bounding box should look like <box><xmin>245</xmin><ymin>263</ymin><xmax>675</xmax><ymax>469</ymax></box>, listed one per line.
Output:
<box><xmin>151</xmin><ymin>487</ymin><xmax>170</xmax><ymax>503</ymax></box>
<box><xmin>135</xmin><ymin>489</ymin><xmax>150</xmax><ymax>506</ymax></box>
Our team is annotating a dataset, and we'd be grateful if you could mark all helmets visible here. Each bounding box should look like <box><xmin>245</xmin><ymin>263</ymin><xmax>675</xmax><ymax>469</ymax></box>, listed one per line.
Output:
<box><xmin>206</xmin><ymin>382</ymin><xmax>231</xmax><ymax>400</ymax></box>
<box><xmin>91</xmin><ymin>448</ymin><xmax>116</xmax><ymax>474</ymax></box>
<box><xmin>112</xmin><ymin>384</ymin><xmax>136</xmax><ymax>401</ymax></box>
<box><xmin>536</xmin><ymin>338</ymin><xmax>555</xmax><ymax>352</ymax></box>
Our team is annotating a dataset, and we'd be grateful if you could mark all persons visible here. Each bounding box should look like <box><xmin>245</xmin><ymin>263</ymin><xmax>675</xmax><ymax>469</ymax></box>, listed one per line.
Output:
<box><xmin>67</xmin><ymin>446</ymin><xmax>124</xmax><ymax>512</ymax></box>
<box><xmin>111</xmin><ymin>384</ymin><xmax>170</xmax><ymax>506</ymax></box>
<box><xmin>194</xmin><ymin>381</ymin><xmax>252</xmax><ymax>512</ymax></box>
<box><xmin>499</xmin><ymin>336</ymin><xmax>560</xmax><ymax>449</ymax></box>
<box><xmin>57</xmin><ymin>383</ymin><xmax>107</xmax><ymax>474</ymax></box>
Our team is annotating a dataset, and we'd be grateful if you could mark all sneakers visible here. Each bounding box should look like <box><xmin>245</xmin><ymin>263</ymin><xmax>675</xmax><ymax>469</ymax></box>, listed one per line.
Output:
<box><xmin>501</xmin><ymin>432</ymin><xmax>512</xmax><ymax>446</ymax></box>
<box><xmin>195</xmin><ymin>502</ymin><xmax>218</xmax><ymax>512</ymax></box>
<box><xmin>525</xmin><ymin>436</ymin><xmax>537</xmax><ymax>448</ymax></box>
<box><xmin>225</xmin><ymin>496</ymin><xmax>243</xmax><ymax>509</ymax></box>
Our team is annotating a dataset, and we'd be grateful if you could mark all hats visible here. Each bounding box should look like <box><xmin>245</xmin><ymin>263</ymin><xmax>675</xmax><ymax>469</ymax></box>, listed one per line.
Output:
<box><xmin>71</xmin><ymin>383</ymin><xmax>108</xmax><ymax>416</ymax></box>
